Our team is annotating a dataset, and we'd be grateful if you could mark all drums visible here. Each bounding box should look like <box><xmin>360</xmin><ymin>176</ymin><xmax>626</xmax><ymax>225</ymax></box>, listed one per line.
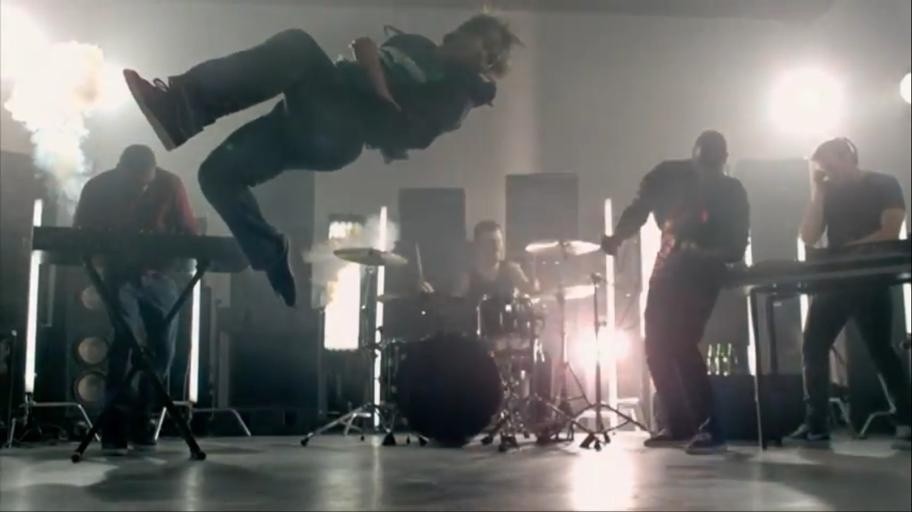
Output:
<box><xmin>384</xmin><ymin>296</ymin><xmax>478</xmax><ymax>337</ymax></box>
<box><xmin>395</xmin><ymin>339</ymin><xmax>502</xmax><ymax>448</ymax></box>
<box><xmin>483</xmin><ymin>297</ymin><xmax>547</xmax><ymax>352</ymax></box>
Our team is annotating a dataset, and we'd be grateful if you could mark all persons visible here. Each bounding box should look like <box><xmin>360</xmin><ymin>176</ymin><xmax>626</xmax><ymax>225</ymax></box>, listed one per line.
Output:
<box><xmin>413</xmin><ymin>218</ymin><xmax>555</xmax><ymax>449</ymax></box>
<box><xmin>598</xmin><ymin>126</ymin><xmax>752</xmax><ymax>458</ymax></box>
<box><xmin>70</xmin><ymin>144</ymin><xmax>201</xmax><ymax>457</ymax></box>
<box><xmin>121</xmin><ymin>11</ymin><xmax>522</xmax><ymax>309</ymax></box>
<box><xmin>778</xmin><ymin>133</ymin><xmax>911</xmax><ymax>447</ymax></box>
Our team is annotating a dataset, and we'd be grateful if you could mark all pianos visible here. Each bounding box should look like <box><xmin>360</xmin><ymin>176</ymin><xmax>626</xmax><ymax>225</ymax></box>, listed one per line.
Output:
<box><xmin>728</xmin><ymin>252</ymin><xmax>908</xmax><ymax>291</ymax></box>
<box><xmin>33</xmin><ymin>227</ymin><xmax>249</xmax><ymax>271</ymax></box>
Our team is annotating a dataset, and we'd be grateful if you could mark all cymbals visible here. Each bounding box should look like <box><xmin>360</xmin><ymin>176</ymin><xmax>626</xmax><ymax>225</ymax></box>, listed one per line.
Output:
<box><xmin>527</xmin><ymin>270</ymin><xmax>601</xmax><ymax>298</ymax></box>
<box><xmin>525</xmin><ymin>239</ymin><xmax>600</xmax><ymax>256</ymax></box>
<box><xmin>335</xmin><ymin>247</ymin><xmax>405</xmax><ymax>265</ymax></box>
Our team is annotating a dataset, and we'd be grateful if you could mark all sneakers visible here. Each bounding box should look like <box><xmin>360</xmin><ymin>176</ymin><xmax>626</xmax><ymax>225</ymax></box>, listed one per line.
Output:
<box><xmin>686</xmin><ymin>434</ymin><xmax>728</xmax><ymax>455</ymax></box>
<box><xmin>123</xmin><ymin>66</ymin><xmax>186</xmax><ymax>149</ymax></box>
<box><xmin>888</xmin><ymin>423</ymin><xmax>910</xmax><ymax>451</ymax></box>
<box><xmin>531</xmin><ymin>414</ymin><xmax>571</xmax><ymax>438</ymax></box>
<box><xmin>782</xmin><ymin>425</ymin><xmax>829</xmax><ymax>443</ymax></box>
<box><xmin>644</xmin><ymin>427</ymin><xmax>693</xmax><ymax>446</ymax></box>
<box><xmin>269</xmin><ymin>231</ymin><xmax>296</xmax><ymax>309</ymax></box>
<box><xmin>132</xmin><ymin>423</ymin><xmax>157</xmax><ymax>450</ymax></box>
<box><xmin>97</xmin><ymin>431</ymin><xmax>128</xmax><ymax>458</ymax></box>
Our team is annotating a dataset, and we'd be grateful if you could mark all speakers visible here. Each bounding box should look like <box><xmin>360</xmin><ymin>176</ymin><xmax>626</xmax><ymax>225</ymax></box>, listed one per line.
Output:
<box><xmin>399</xmin><ymin>188</ymin><xmax>464</xmax><ymax>252</ymax></box>
<box><xmin>32</xmin><ymin>262</ymin><xmax>117</xmax><ymax>419</ymax></box>
<box><xmin>505</xmin><ymin>173</ymin><xmax>577</xmax><ymax>259</ymax></box>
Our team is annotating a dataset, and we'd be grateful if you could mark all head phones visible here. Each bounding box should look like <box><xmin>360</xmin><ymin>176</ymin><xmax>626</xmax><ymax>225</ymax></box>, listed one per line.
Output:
<box><xmin>814</xmin><ymin>136</ymin><xmax>859</xmax><ymax>166</ymax></box>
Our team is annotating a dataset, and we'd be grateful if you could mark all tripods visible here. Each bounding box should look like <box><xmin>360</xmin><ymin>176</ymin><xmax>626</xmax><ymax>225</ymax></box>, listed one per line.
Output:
<box><xmin>480</xmin><ymin>261</ymin><xmax>659</xmax><ymax>451</ymax></box>
<box><xmin>299</xmin><ymin>263</ymin><xmax>431</xmax><ymax>446</ymax></box>
